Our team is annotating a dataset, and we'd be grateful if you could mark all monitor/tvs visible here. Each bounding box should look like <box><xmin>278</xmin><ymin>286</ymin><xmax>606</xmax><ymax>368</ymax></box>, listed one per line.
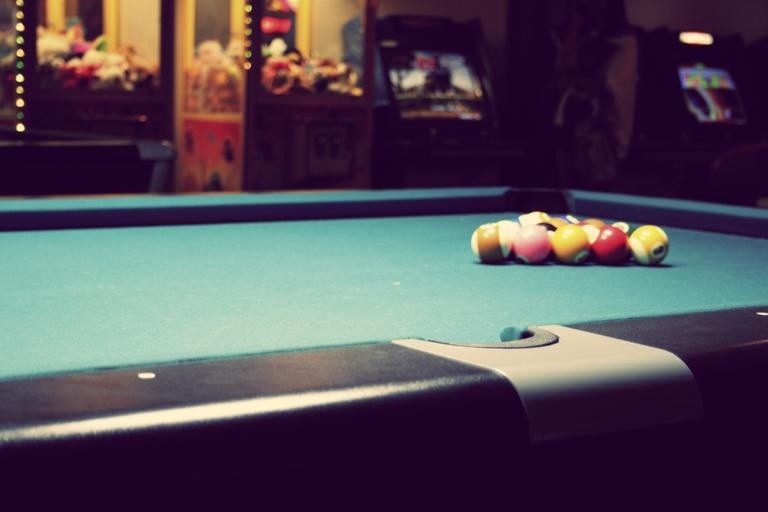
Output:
<box><xmin>672</xmin><ymin>60</ymin><xmax>746</xmax><ymax>124</ymax></box>
<box><xmin>383</xmin><ymin>45</ymin><xmax>487</xmax><ymax>123</ymax></box>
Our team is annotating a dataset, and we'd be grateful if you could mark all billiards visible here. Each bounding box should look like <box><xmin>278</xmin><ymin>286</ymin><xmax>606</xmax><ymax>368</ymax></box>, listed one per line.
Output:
<box><xmin>471</xmin><ymin>210</ymin><xmax>670</xmax><ymax>266</ymax></box>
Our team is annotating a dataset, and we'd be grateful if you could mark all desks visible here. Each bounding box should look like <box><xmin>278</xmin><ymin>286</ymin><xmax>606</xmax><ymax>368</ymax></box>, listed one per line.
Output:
<box><xmin>0</xmin><ymin>183</ymin><xmax>768</xmax><ymax>512</ymax></box>
